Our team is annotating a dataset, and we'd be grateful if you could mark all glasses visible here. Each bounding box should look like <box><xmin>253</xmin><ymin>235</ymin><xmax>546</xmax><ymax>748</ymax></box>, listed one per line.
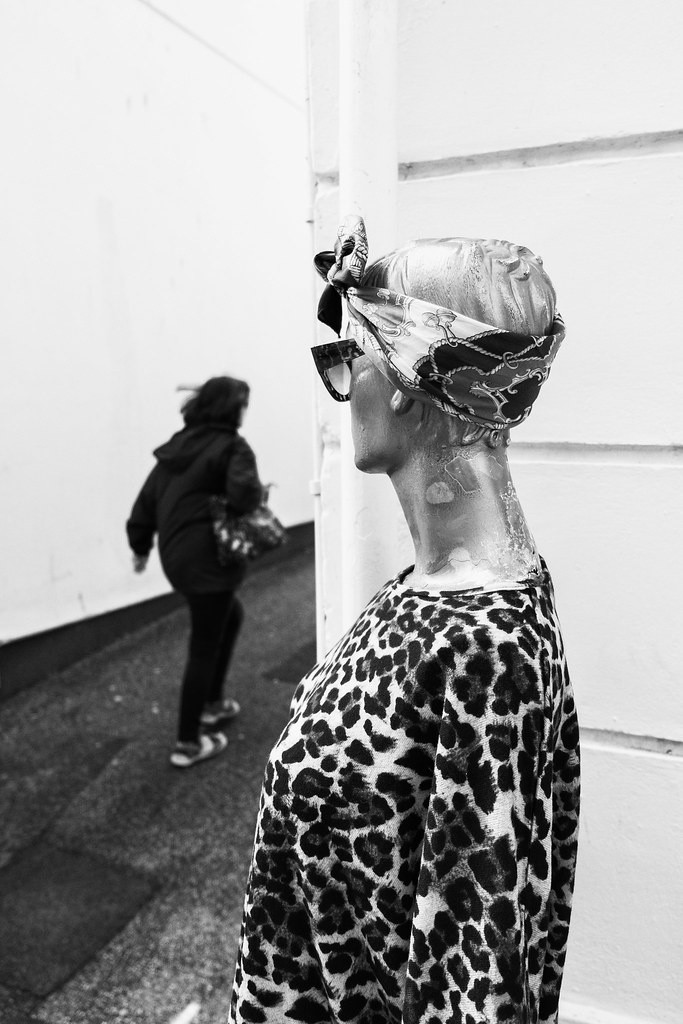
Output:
<box><xmin>310</xmin><ymin>338</ymin><xmax>365</xmax><ymax>402</ymax></box>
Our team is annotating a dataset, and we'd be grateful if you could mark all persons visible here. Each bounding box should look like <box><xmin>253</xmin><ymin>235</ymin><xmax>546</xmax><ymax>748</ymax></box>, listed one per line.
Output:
<box><xmin>126</xmin><ymin>377</ymin><xmax>270</xmax><ymax>766</ymax></box>
<box><xmin>228</xmin><ymin>219</ymin><xmax>581</xmax><ymax>1024</ymax></box>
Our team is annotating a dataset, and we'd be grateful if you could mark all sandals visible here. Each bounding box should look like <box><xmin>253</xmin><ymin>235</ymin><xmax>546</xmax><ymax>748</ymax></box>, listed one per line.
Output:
<box><xmin>169</xmin><ymin>730</ymin><xmax>229</xmax><ymax>767</ymax></box>
<box><xmin>200</xmin><ymin>696</ymin><xmax>240</xmax><ymax>725</ymax></box>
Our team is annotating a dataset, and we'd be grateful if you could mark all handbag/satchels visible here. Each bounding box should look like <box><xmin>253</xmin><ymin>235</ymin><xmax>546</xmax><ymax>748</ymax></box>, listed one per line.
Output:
<box><xmin>210</xmin><ymin>479</ymin><xmax>290</xmax><ymax>566</ymax></box>
